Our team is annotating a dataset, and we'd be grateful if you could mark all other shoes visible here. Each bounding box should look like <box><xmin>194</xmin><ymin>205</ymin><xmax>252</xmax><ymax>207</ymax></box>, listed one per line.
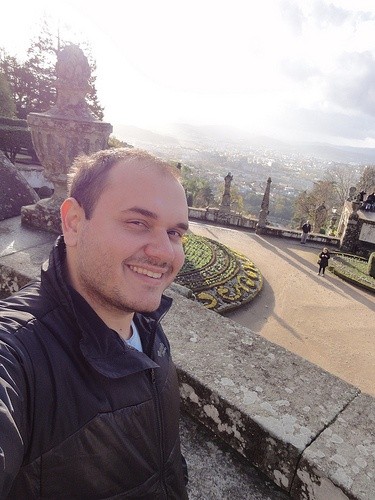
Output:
<box><xmin>317</xmin><ymin>273</ymin><xmax>320</xmax><ymax>275</ymax></box>
<box><xmin>321</xmin><ymin>274</ymin><xmax>324</xmax><ymax>277</ymax></box>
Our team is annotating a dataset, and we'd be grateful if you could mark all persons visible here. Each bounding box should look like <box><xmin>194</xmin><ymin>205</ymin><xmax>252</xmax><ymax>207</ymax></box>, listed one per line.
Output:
<box><xmin>318</xmin><ymin>248</ymin><xmax>330</xmax><ymax>277</ymax></box>
<box><xmin>1</xmin><ymin>147</ymin><xmax>189</xmax><ymax>499</ymax></box>
<box><xmin>300</xmin><ymin>221</ymin><xmax>311</xmax><ymax>244</ymax></box>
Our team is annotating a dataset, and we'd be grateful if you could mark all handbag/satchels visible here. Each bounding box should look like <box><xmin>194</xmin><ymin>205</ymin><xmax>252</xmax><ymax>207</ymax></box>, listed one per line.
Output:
<box><xmin>317</xmin><ymin>260</ymin><xmax>321</xmax><ymax>265</ymax></box>
